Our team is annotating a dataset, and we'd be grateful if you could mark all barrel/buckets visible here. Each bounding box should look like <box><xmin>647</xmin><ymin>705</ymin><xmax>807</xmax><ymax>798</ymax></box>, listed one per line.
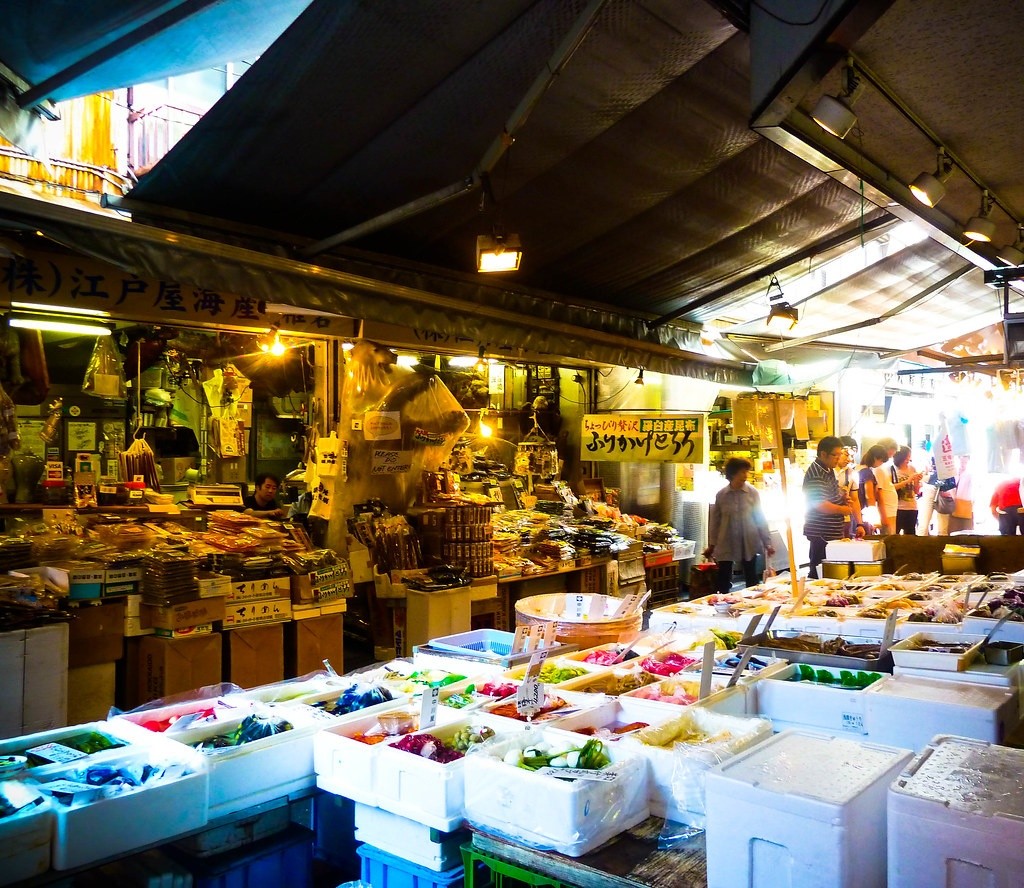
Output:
<box><xmin>515</xmin><ymin>592</ymin><xmax>643</xmax><ymax>649</ymax></box>
<box><xmin>688</xmin><ymin>551</ymin><xmax>720</xmax><ymax>598</ymax></box>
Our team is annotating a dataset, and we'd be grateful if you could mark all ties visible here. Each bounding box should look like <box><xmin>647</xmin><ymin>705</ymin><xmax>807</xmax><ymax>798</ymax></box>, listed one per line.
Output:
<box><xmin>955</xmin><ymin>474</ymin><xmax>961</xmax><ymax>494</ymax></box>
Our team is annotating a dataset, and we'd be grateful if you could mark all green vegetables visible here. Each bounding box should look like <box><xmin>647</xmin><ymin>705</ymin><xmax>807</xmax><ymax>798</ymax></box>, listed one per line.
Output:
<box><xmin>514</xmin><ymin>664</ymin><xmax>588</xmax><ymax>685</ymax></box>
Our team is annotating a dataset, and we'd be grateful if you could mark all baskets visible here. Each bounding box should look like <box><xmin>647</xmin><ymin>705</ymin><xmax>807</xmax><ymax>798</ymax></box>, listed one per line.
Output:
<box><xmin>418</xmin><ymin>628</ymin><xmax>561</xmax><ymax>669</ymax></box>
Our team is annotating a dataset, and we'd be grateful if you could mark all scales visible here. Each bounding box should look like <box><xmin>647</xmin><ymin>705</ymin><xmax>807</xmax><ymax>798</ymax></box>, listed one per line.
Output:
<box><xmin>186</xmin><ymin>480</ymin><xmax>244</xmax><ymax>506</ymax></box>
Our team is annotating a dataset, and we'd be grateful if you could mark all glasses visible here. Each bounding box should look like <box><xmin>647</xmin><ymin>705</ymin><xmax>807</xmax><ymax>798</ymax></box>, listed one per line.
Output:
<box><xmin>827</xmin><ymin>452</ymin><xmax>842</xmax><ymax>459</ymax></box>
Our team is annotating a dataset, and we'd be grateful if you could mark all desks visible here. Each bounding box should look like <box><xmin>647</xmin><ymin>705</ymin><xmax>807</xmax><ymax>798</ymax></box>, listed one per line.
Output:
<box><xmin>645</xmin><ymin>561</ymin><xmax>680</xmax><ymax>611</ymax></box>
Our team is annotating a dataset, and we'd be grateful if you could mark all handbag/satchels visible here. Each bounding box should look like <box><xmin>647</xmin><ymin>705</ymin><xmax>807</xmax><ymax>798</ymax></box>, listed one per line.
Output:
<box><xmin>118</xmin><ymin>438</ymin><xmax>163</xmax><ymax>495</ymax></box>
<box><xmin>212</xmin><ymin>408</ymin><xmax>247</xmax><ymax>459</ymax></box>
<box><xmin>891</xmin><ymin>465</ymin><xmax>901</xmax><ymax>500</ymax></box>
<box><xmin>952</xmin><ymin>497</ymin><xmax>972</xmax><ymax>519</ymax></box>
<box><xmin>928</xmin><ymin>457</ymin><xmax>956</xmax><ymax>491</ymax></box>
<box><xmin>935</xmin><ymin>492</ymin><xmax>955</xmax><ymax>514</ymax></box>
<box><xmin>689</xmin><ymin>553</ymin><xmax>720</xmax><ymax>601</ymax></box>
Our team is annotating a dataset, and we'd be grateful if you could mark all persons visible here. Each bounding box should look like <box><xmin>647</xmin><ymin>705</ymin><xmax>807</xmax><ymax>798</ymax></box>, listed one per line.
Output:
<box><xmin>802</xmin><ymin>435</ymin><xmax>1024</xmax><ymax>580</ymax></box>
<box><xmin>702</xmin><ymin>458</ymin><xmax>775</xmax><ymax>595</ymax></box>
<box><xmin>244</xmin><ymin>473</ymin><xmax>284</xmax><ymax>521</ymax></box>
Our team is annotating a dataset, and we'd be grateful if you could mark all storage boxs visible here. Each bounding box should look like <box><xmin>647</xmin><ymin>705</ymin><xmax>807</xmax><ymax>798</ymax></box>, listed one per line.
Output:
<box><xmin>557</xmin><ymin>557</ymin><xmax>580</xmax><ymax>570</ymax></box>
<box><xmin>212</xmin><ymin>455</ymin><xmax>246</xmax><ymax>483</ymax></box>
<box><xmin>40</xmin><ymin>561</ymin><xmax>105</xmax><ymax>598</ymax></box>
<box><xmin>568</xmin><ymin>567</ymin><xmax>602</xmax><ymax>593</ymax></box>
<box><xmin>577</xmin><ymin>477</ymin><xmax>607</xmax><ymax>502</ymax></box>
<box><xmin>0</xmin><ymin>584</ymin><xmax>1024</xmax><ymax>888</ymax></box>
<box><xmin>102</xmin><ymin>567</ymin><xmax>143</xmax><ymax>598</ymax></box>
<box><xmin>617</xmin><ymin>541</ymin><xmax>646</xmax><ymax>581</ymax></box>
<box><xmin>373</xmin><ymin>564</ymin><xmax>433</xmax><ymax>598</ymax></box>
<box><xmin>291</xmin><ymin>557</ymin><xmax>355</xmax><ymax>605</ymax></box>
<box><xmin>644</xmin><ymin>539</ymin><xmax>697</xmax><ymax>568</ymax></box>
<box><xmin>193</xmin><ymin>572</ymin><xmax>231</xmax><ymax>598</ymax></box>
<box><xmin>154</xmin><ymin>457</ymin><xmax>195</xmax><ymax>485</ymax></box>
<box><xmin>822</xmin><ymin>561</ymin><xmax>852</xmax><ymax>580</ymax></box>
<box><xmin>941</xmin><ymin>553</ymin><xmax>976</xmax><ymax>574</ymax></box>
<box><xmin>223</xmin><ymin>577</ymin><xmax>290</xmax><ymax>604</ymax></box>
<box><xmin>854</xmin><ymin>558</ymin><xmax>887</xmax><ymax>577</ymax></box>
<box><xmin>825</xmin><ymin>542</ymin><xmax>886</xmax><ymax>561</ymax></box>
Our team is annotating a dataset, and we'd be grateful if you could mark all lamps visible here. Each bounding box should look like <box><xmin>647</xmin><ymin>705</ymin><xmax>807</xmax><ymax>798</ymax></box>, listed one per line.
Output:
<box><xmin>572</xmin><ymin>375</ymin><xmax>582</xmax><ymax>383</ymax></box>
<box><xmin>256</xmin><ymin>322</ymin><xmax>281</xmax><ymax>352</ymax></box>
<box><xmin>3</xmin><ymin>312</ymin><xmax>116</xmax><ymax>336</ymax></box>
<box><xmin>475</xmin><ymin>230</ymin><xmax>524</xmax><ymax>275</ymax></box>
<box><xmin>766</xmin><ymin>303</ymin><xmax>800</xmax><ymax>331</ymax></box>
<box><xmin>996</xmin><ymin>224</ymin><xmax>1024</xmax><ymax>269</ymax></box>
<box><xmin>473</xmin><ymin>346</ymin><xmax>488</xmax><ymax>371</ymax></box>
<box><xmin>908</xmin><ymin>147</ymin><xmax>956</xmax><ymax>208</ymax></box>
<box><xmin>962</xmin><ymin>189</ymin><xmax>996</xmax><ymax>242</ymax></box>
<box><xmin>634</xmin><ymin>369</ymin><xmax>644</xmax><ymax>388</ymax></box>
<box><xmin>810</xmin><ymin>56</ymin><xmax>864</xmax><ymax>139</ymax></box>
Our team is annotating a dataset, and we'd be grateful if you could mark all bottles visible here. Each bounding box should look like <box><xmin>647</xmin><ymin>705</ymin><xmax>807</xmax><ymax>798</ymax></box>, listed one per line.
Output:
<box><xmin>0</xmin><ymin>447</ymin><xmax>45</xmax><ymax>506</ymax></box>
